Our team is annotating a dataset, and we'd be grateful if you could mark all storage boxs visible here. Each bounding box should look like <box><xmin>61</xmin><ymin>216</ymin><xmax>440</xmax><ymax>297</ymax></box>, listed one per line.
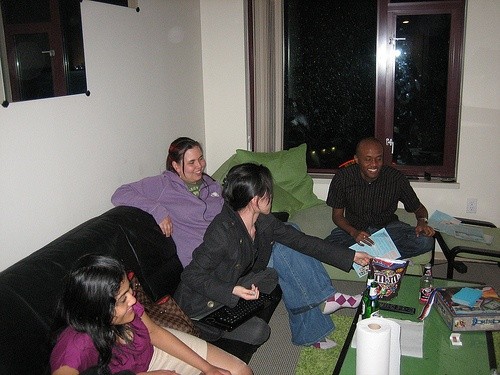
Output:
<box><xmin>433</xmin><ymin>285</ymin><xmax>500</xmax><ymax>331</ymax></box>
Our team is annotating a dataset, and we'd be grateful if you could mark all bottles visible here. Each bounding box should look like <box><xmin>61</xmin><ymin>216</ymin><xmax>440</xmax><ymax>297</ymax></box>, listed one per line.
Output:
<box><xmin>418</xmin><ymin>263</ymin><xmax>435</xmax><ymax>305</ymax></box>
<box><xmin>364</xmin><ymin>264</ymin><xmax>375</xmax><ymax>289</ymax></box>
<box><xmin>361</xmin><ymin>282</ymin><xmax>381</xmax><ymax>320</ymax></box>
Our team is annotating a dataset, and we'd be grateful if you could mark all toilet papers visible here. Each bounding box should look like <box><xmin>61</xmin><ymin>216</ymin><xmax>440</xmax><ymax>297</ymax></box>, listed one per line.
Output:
<box><xmin>356</xmin><ymin>317</ymin><xmax>400</xmax><ymax>375</ymax></box>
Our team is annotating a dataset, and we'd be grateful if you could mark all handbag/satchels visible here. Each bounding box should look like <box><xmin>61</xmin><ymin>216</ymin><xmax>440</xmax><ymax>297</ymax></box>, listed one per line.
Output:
<box><xmin>124</xmin><ymin>271</ymin><xmax>154</xmax><ymax>312</ymax></box>
<box><xmin>131</xmin><ymin>289</ymin><xmax>202</xmax><ymax>340</ymax></box>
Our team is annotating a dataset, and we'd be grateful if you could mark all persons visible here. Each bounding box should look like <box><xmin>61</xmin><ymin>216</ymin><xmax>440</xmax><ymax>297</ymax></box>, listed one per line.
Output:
<box><xmin>47</xmin><ymin>253</ymin><xmax>254</xmax><ymax>375</ymax></box>
<box><xmin>173</xmin><ymin>163</ymin><xmax>375</xmax><ymax>345</ymax></box>
<box><xmin>325</xmin><ymin>137</ymin><xmax>435</xmax><ymax>261</ymax></box>
<box><xmin>112</xmin><ymin>137</ymin><xmax>363</xmax><ymax>350</ymax></box>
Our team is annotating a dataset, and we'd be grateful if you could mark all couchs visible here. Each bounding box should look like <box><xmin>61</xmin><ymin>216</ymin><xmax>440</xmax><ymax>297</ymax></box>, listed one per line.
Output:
<box><xmin>0</xmin><ymin>204</ymin><xmax>431</xmax><ymax>375</ymax></box>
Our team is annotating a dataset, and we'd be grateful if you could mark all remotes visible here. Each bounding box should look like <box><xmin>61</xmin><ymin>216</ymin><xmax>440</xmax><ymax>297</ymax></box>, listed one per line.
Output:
<box><xmin>377</xmin><ymin>301</ymin><xmax>416</xmax><ymax>315</ymax></box>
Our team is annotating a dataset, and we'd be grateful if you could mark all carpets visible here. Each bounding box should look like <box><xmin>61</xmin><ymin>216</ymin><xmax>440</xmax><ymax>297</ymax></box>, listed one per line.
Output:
<box><xmin>296</xmin><ymin>315</ymin><xmax>500</xmax><ymax>375</ymax></box>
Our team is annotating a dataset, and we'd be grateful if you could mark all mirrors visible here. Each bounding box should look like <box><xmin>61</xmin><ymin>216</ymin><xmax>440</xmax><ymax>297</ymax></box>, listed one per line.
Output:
<box><xmin>0</xmin><ymin>0</ymin><xmax>87</xmax><ymax>102</ymax></box>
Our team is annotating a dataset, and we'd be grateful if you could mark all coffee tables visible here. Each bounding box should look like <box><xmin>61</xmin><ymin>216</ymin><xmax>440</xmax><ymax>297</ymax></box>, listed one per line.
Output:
<box><xmin>332</xmin><ymin>274</ymin><xmax>497</xmax><ymax>375</ymax></box>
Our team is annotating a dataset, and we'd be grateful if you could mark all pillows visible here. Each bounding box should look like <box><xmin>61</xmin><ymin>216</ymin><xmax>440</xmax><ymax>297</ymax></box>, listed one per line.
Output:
<box><xmin>210</xmin><ymin>142</ymin><xmax>326</xmax><ymax>219</ymax></box>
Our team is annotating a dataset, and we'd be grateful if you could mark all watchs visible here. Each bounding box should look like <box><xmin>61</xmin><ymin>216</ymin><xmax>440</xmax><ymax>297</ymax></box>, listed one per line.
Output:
<box><xmin>417</xmin><ymin>217</ymin><xmax>428</xmax><ymax>224</ymax></box>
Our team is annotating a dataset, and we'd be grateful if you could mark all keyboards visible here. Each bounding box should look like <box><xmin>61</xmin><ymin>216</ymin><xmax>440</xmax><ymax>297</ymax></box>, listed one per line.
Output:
<box><xmin>199</xmin><ymin>293</ymin><xmax>279</xmax><ymax>333</ymax></box>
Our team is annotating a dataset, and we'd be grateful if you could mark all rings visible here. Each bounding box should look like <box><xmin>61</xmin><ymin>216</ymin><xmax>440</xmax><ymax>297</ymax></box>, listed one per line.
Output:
<box><xmin>363</xmin><ymin>235</ymin><xmax>365</xmax><ymax>238</ymax></box>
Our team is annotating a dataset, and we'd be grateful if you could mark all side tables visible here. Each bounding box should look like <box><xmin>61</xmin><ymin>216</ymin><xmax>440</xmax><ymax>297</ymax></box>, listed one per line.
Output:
<box><xmin>433</xmin><ymin>217</ymin><xmax>500</xmax><ymax>280</ymax></box>
